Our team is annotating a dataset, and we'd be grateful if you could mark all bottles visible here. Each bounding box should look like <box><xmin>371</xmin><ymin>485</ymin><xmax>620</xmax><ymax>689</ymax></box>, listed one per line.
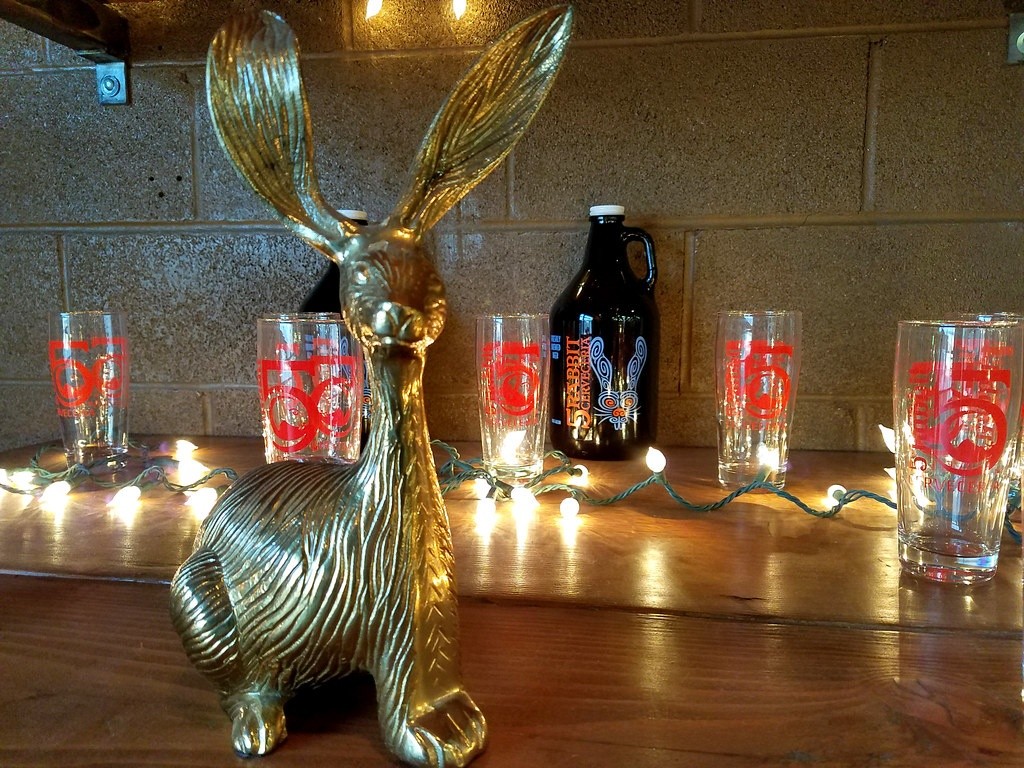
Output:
<box><xmin>547</xmin><ymin>205</ymin><xmax>660</xmax><ymax>461</ymax></box>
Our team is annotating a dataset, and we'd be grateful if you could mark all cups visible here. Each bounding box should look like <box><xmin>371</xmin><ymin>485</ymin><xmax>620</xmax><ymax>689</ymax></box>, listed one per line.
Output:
<box><xmin>892</xmin><ymin>311</ymin><xmax>1024</xmax><ymax>588</ymax></box>
<box><xmin>715</xmin><ymin>310</ymin><xmax>803</xmax><ymax>494</ymax></box>
<box><xmin>474</xmin><ymin>311</ymin><xmax>550</xmax><ymax>479</ymax></box>
<box><xmin>48</xmin><ymin>310</ymin><xmax>130</xmax><ymax>475</ymax></box>
<box><xmin>256</xmin><ymin>312</ymin><xmax>366</xmax><ymax>463</ymax></box>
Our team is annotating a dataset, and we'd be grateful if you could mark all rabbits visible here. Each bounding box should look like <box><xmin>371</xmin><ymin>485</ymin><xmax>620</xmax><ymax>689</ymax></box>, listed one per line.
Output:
<box><xmin>164</xmin><ymin>4</ymin><xmax>575</xmax><ymax>768</ymax></box>
